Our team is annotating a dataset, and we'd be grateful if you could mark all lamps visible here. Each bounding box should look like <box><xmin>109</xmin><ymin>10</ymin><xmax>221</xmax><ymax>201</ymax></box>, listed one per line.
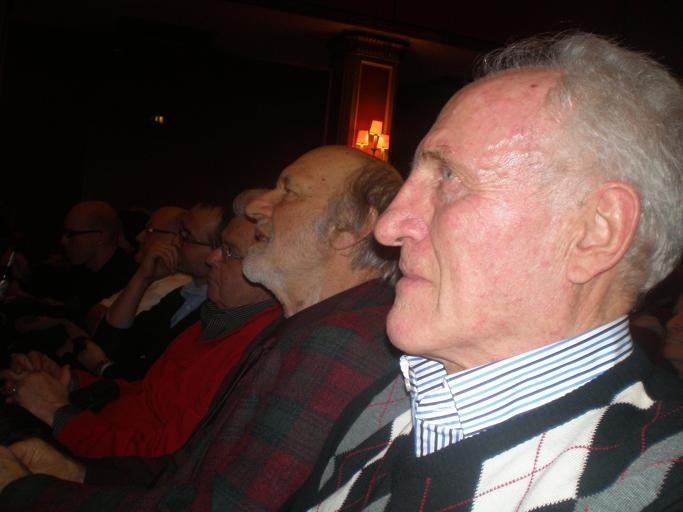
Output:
<box><xmin>355</xmin><ymin>117</ymin><xmax>390</xmax><ymax>160</ymax></box>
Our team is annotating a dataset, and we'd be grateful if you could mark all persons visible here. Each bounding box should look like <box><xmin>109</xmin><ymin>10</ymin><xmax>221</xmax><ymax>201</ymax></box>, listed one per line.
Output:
<box><xmin>2</xmin><ymin>145</ymin><xmax>406</xmax><ymax>511</ymax></box>
<box><xmin>275</xmin><ymin>35</ymin><xmax>682</xmax><ymax>511</ymax></box>
<box><xmin>629</xmin><ymin>258</ymin><xmax>681</xmax><ymax>380</ymax></box>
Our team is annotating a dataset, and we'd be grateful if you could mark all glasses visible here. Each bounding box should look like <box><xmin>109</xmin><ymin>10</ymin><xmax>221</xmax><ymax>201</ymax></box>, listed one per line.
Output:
<box><xmin>142</xmin><ymin>226</ymin><xmax>177</xmax><ymax>234</ymax></box>
<box><xmin>219</xmin><ymin>243</ymin><xmax>245</xmax><ymax>261</ymax></box>
<box><xmin>65</xmin><ymin>229</ymin><xmax>94</xmax><ymax>235</ymax></box>
<box><xmin>179</xmin><ymin>229</ymin><xmax>213</xmax><ymax>247</ymax></box>
